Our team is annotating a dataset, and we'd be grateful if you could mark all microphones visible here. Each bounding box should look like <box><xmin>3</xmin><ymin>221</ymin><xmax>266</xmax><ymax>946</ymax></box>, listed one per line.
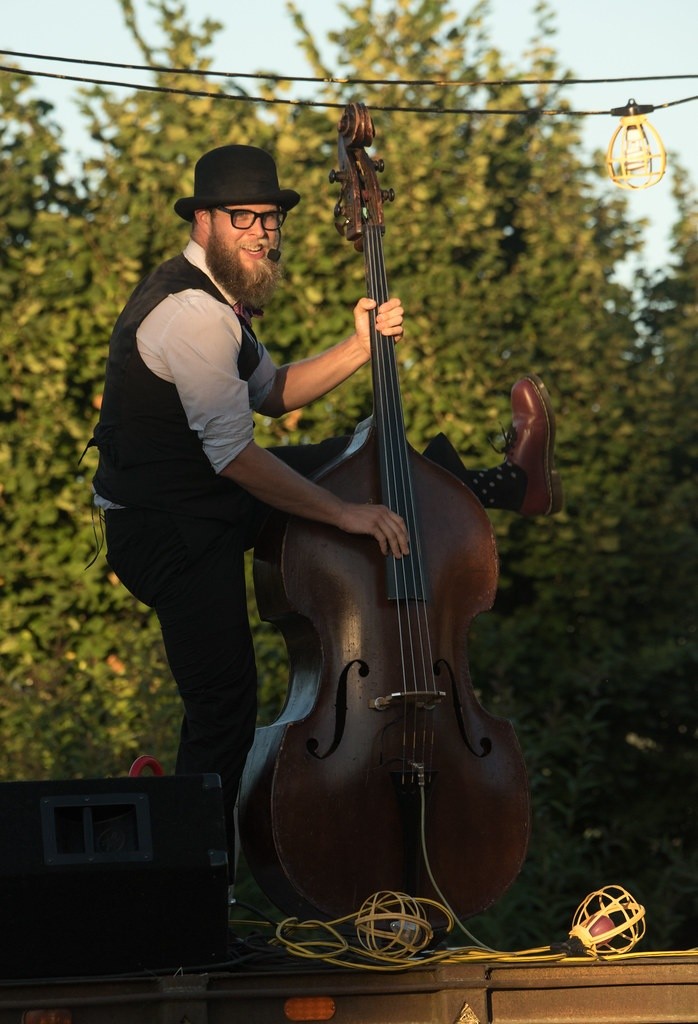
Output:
<box><xmin>267</xmin><ymin>230</ymin><xmax>281</xmax><ymax>263</ymax></box>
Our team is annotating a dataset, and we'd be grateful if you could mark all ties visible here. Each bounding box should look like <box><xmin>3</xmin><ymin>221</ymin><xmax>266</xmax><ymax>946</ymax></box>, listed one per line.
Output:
<box><xmin>233</xmin><ymin>300</ymin><xmax>252</xmax><ymax>327</ymax></box>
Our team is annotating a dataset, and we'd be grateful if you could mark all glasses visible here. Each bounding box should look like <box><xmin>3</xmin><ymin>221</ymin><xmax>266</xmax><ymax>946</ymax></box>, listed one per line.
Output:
<box><xmin>217</xmin><ymin>206</ymin><xmax>287</xmax><ymax>230</ymax></box>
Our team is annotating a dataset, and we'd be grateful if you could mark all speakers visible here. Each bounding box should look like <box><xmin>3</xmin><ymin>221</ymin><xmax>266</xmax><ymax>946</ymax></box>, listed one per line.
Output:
<box><xmin>0</xmin><ymin>772</ymin><xmax>230</xmax><ymax>980</ymax></box>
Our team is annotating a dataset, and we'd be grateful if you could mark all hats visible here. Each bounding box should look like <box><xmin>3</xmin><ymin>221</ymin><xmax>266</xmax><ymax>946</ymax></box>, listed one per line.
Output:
<box><xmin>173</xmin><ymin>145</ymin><xmax>300</xmax><ymax>221</ymax></box>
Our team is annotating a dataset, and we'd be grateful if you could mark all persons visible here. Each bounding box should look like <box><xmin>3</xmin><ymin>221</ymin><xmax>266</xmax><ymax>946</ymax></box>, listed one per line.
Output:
<box><xmin>92</xmin><ymin>145</ymin><xmax>564</xmax><ymax>972</ymax></box>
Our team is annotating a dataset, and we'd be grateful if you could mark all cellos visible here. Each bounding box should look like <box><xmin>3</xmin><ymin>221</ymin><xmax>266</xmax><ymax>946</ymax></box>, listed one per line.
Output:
<box><xmin>237</xmin><ymin>103</ymin><xmax>532</xmax><ymax>943</ymax></box>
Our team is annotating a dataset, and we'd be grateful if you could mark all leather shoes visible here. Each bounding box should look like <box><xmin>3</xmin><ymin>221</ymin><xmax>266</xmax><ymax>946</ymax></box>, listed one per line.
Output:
<box><xmin>487</xmin><ymin>374</ymin><xmax>563</xmax><ymax>517</ymax></box>
